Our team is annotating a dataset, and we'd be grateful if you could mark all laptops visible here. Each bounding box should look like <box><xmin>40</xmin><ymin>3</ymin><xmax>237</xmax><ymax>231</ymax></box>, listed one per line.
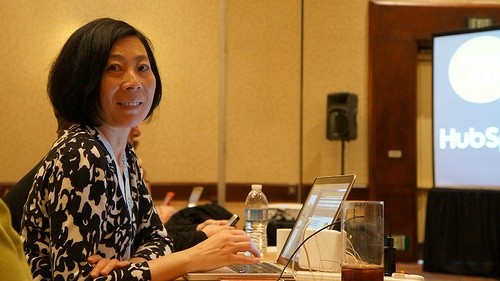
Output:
<box><xmin>183</xmin><ymin>174</ymin><xmax>356</xmax><ymax>280</ymax></box>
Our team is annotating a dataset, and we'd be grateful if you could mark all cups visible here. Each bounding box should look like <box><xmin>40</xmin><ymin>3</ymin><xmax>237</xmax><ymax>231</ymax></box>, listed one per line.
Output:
<box><xmin>340</xmin><ymin>200</ymin><xmax>385</xmax><ymax>281</ymax></box>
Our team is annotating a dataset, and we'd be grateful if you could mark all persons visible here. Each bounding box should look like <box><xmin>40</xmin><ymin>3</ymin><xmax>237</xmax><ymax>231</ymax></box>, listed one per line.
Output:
<box><xmin>2</xmin><ymin>108</ymin><xmax>237</xmax><ymax>253</ymax></box>
<box><xmin>21</xmin><ymin>18</ymin><xmax>262</xmax><ymax>281</ymax></box>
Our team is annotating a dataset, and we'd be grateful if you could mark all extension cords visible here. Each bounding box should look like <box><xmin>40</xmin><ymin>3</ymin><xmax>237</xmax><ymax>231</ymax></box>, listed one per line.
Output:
<box><xmin>292</xmin><ymin>271</ymin><xmax>424</xmax><ymax>281</ymax></box>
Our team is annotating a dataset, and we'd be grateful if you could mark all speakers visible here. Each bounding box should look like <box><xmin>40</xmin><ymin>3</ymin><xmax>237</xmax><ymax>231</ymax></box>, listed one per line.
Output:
<box><xmin>326</xmin><ymin>92</ymin><xmax>358</xmax><ymax>141</ymax></box>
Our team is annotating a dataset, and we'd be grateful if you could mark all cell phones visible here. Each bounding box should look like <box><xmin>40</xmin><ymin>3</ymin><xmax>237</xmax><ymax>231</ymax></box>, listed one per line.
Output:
<box><xmin>227</xmin><ymin>214</ymin><xmax>239</xmax><ymax>227</ymax></box>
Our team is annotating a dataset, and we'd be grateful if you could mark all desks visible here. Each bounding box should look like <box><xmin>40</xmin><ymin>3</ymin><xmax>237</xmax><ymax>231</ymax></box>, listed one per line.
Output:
<box><xmin>217</xmin><ymin>252</ymin><xmax>497</xmax><ymax>281</ymax></box>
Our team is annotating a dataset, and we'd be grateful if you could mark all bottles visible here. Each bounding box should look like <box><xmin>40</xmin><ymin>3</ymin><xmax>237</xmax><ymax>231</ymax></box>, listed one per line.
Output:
<box><xmin>245</xmin><ymin>184</ymin><xmax>269</xmax><ymax>262</ymax></box>
